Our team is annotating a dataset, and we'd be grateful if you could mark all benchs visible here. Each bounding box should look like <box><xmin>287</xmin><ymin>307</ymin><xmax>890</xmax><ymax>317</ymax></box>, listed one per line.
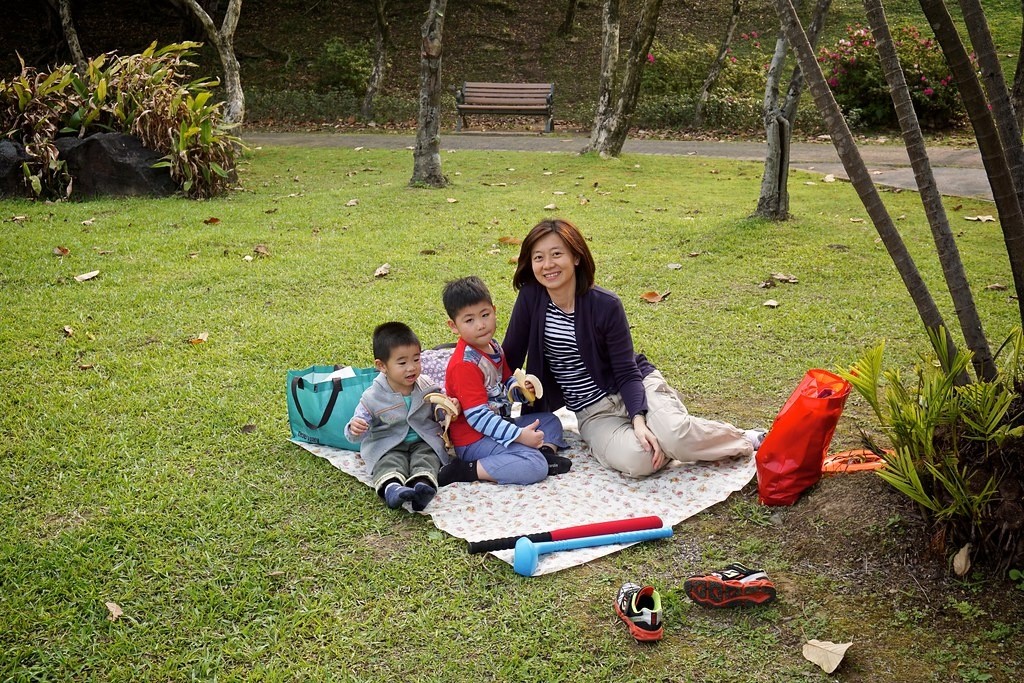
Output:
<box><xmin>456</xmin><ymin>81</ymin><xmax>555</xmax><ymax>133</ymax></box>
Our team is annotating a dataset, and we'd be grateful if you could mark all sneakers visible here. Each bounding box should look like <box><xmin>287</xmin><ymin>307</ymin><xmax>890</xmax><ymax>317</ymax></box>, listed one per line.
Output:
<box><xmin>614</xmin><ymin>581</ymin><xmax>665</xmax><ymax>641</ymax></box>
<box><xmin>684</xmin><ymin>562</ymin><xmax>776</xmax><ymax>608</ymax></box>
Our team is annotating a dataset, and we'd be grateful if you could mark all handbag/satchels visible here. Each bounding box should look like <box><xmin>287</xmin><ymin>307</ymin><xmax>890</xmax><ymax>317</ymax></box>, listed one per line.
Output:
<box><xmin>755</xmin><ymin>366</ymin><xmax>859</xmax><ymax>507</ymax></box>
<box><xmin>286</xmin><ymin>364</ymin><xmax>380</xmax><ymax>451</ymax></box>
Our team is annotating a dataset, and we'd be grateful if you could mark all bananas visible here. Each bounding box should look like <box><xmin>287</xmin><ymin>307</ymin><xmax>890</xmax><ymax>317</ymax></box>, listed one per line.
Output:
<box><xmin>508</xmin><ymin>367</ymin><xmax>543</xmax><ymax>402</ymax></box>
<box><xmin>423</xmin><ymin>392</ymin><xmax>459</xmax><ymax>448</ymax></box>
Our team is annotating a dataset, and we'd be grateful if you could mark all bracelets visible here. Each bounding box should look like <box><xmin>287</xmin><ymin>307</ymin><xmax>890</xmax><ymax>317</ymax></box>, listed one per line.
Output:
<box><xmin>632</xmin><ymin>414</ymin><xmax>647</xmax><ymax>426</ymax></box>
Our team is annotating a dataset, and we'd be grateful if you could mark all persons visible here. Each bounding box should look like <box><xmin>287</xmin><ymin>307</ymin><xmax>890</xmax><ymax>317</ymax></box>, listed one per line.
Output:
<box><xmin>436</xmin><ymin>276</ymin><xmax>573</xmax><ymax>488</ymax></box>
<box><xmin>342</xmin><ymin>321</ymin><xmax>461</xmax><ymax>512</ymax></box>
<box><xmin>501</xmin><ymin>219</ymin><xmax>768</xmax><ymax>476</ymax></box>
